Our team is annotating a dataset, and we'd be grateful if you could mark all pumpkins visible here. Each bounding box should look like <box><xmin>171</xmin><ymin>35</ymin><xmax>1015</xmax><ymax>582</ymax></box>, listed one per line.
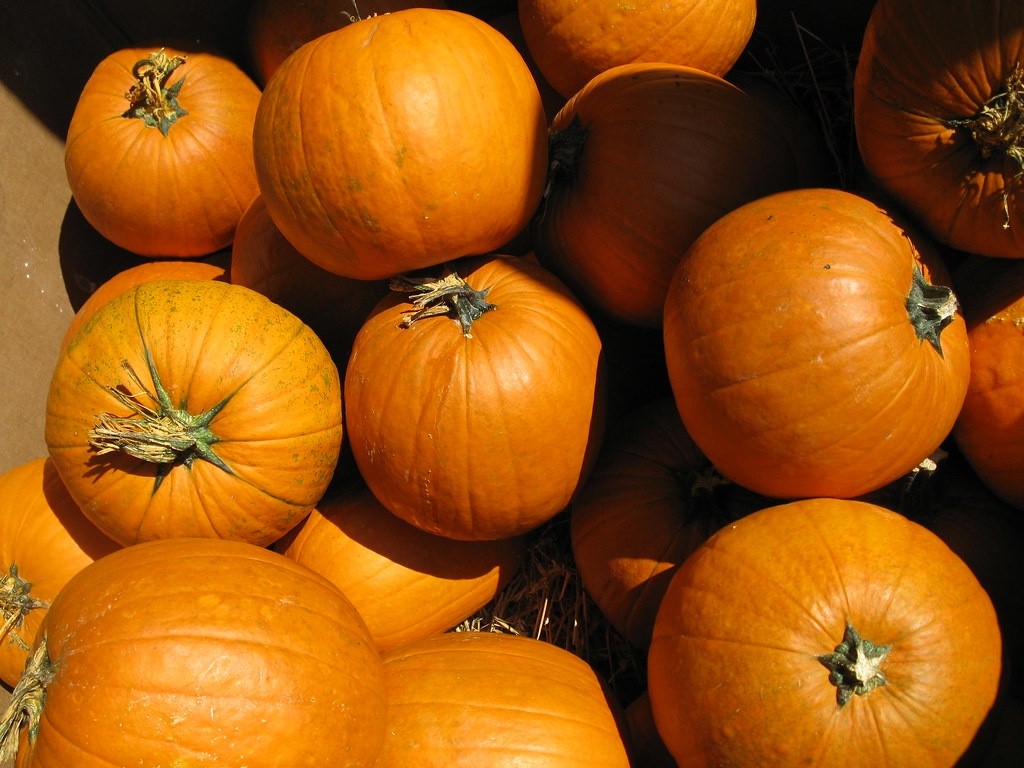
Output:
<box><xmin>1</xmin><ymin>0</ymin><xmax>1024</xmax><ymax>768</ymax></box>
